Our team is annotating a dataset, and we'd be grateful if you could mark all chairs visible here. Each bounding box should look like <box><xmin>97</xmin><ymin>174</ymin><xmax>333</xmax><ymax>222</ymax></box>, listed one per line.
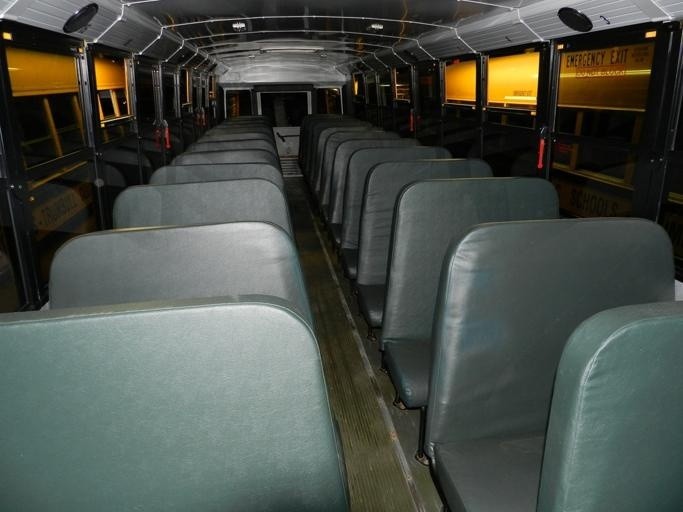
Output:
<box><xmin>0</xmin><ymin>118</ymin><xmax>683</xmax><ymax>512</ymax></box>
